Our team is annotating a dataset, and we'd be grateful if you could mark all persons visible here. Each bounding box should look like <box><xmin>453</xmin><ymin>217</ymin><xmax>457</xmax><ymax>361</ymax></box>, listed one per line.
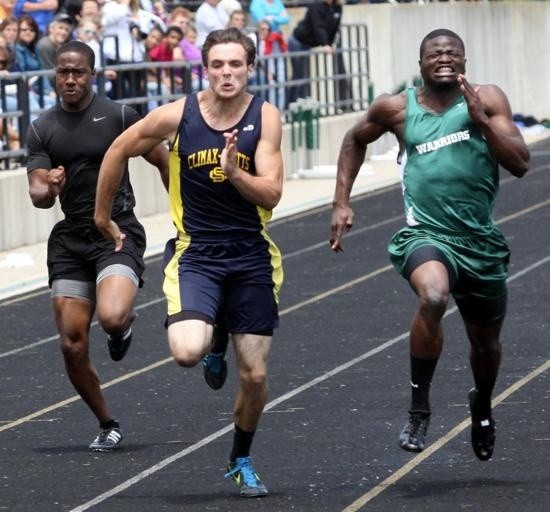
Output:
<box><xmin>0</xmin><ymin>0</ymin><xmax>346</xmax><ymax>167</ymax></box>
<box><xmin>92</xmin><ymin>27</ymin><xmax>285</xmax><ymax>497</ymax></box>
<box><xmin>26</xmin><ymin>43</ymin><xmax>171</xmax><ymax>457</ymax></box>
<box><xmin>329</xmin><ymin>28</ymin><xmax>529</xmax><ymax>462</ymax></box>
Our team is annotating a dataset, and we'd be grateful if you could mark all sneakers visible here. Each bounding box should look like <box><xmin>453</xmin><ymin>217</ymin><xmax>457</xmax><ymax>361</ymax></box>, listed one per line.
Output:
<box><xmin>107</xmin><ymin>326</ymin><xmax>132</xmax><ymax>362</ymax></box>
<box><xmin>227</xmin><ymin>456</ymin><xmax>268</xmax><ymax>497</ymax></box>
<box><xmin>202</xmin><ymin>326</ymin><xmax>229</xmax><ymax>389</ymax></box>
<box><xmin>469</xmin><ymin>388</ymin><xmax>495</xmax><ymax>460</ymax></box>
<box><xmin>88</xmin><ymin>429</ymin><xmax>123</xmax><ymax>451</ymax></box>
<box><xmin>400</xmin><ymin>410</ymin><xmax>430</xmax><ymax>452</ymax></box>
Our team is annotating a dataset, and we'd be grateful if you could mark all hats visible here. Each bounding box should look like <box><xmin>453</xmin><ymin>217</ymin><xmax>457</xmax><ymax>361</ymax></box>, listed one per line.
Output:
<box><xmin>50</xmin><ymin>14</ymin><xmax>76</xmax><ymax>26</ymax></box>
